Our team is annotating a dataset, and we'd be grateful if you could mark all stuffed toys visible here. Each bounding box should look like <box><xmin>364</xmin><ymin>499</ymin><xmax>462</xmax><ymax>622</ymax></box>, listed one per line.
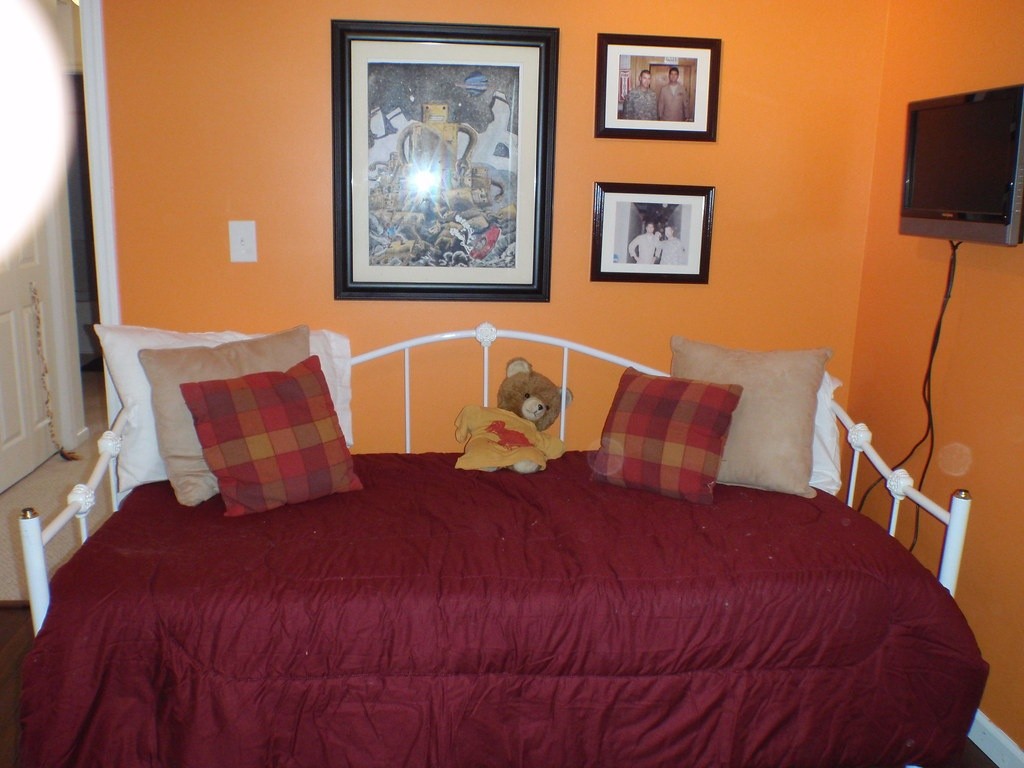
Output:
<box><xmin>454</xmin><ymin>358</ymin><xmax>573</xmax><ymax>474</ymax></box>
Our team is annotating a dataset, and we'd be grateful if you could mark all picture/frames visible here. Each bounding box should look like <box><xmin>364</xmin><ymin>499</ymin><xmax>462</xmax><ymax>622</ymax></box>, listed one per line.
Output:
<box><xmin>594</xmin><ymin>32</ymin><xmax>720</xmax><ymax>142</ymax></box>
<box><xmin>589</xmin><ymin>180</ymin><xmax>716</xmax><ymax>284</ymax></box>
<box><xmin>328</xmin><ymin>18</ymin><xmax>560</xmax><ymax>305</ymax></box>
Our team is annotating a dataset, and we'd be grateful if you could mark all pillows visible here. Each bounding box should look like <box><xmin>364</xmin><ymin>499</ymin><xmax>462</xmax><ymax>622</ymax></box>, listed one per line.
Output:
<box><xmin>178</xmin><ymin>354</ymin><xmax>363</xmax><ymax>515</ymax></box>
<box><xmin>138</xmin><ymin>323</ymin><xmax>310</xmax><ymax>508</ymax></box>
<box><xmin>662</xmin><ymin>331</ymin><xmax>833</xmax><ymax>500</ymax></box>
<box><xmin>807</xmin><ymin>372</ymin><xmax>843</xmax><ymax>496</ymax></box>
<box><xmin>93</xmin><ymin>323</ymin><xmax>353</xmax><ymax>491</ymax></box>
<box><xmin>589</xmin><ymin>365</ymin><xmax>742</xmax><ymax>505</ymax></box>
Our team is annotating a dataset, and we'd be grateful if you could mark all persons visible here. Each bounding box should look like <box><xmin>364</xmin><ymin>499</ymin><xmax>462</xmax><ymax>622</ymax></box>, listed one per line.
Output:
<box><xmin>622</xmin><ymin>70</ymin><xmax>658</xmax><ymax>120</ymax></box>
<box><xmin>629</xmin><ymin>222</ymin><xmax>682</xmax><ymax>265</ymax></box>
<box><xmin>658</xmin><ymin>67</ymin><xmax>692</xmax><ymax>122</ymax></box>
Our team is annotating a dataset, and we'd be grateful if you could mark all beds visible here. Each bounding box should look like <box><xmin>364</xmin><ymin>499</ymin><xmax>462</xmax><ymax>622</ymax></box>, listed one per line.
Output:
<box><xmin>22</xmin><ymin>324</ymin><xmax>1023</xmax><ymax>767</ymax></box>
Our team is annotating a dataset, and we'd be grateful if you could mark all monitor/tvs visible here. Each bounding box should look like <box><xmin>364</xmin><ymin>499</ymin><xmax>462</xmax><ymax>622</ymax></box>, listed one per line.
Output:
<box><xmin>900</xmin><ymin>85</ymin><xmax>1024</xmax><ymax>248</ymax></box>
